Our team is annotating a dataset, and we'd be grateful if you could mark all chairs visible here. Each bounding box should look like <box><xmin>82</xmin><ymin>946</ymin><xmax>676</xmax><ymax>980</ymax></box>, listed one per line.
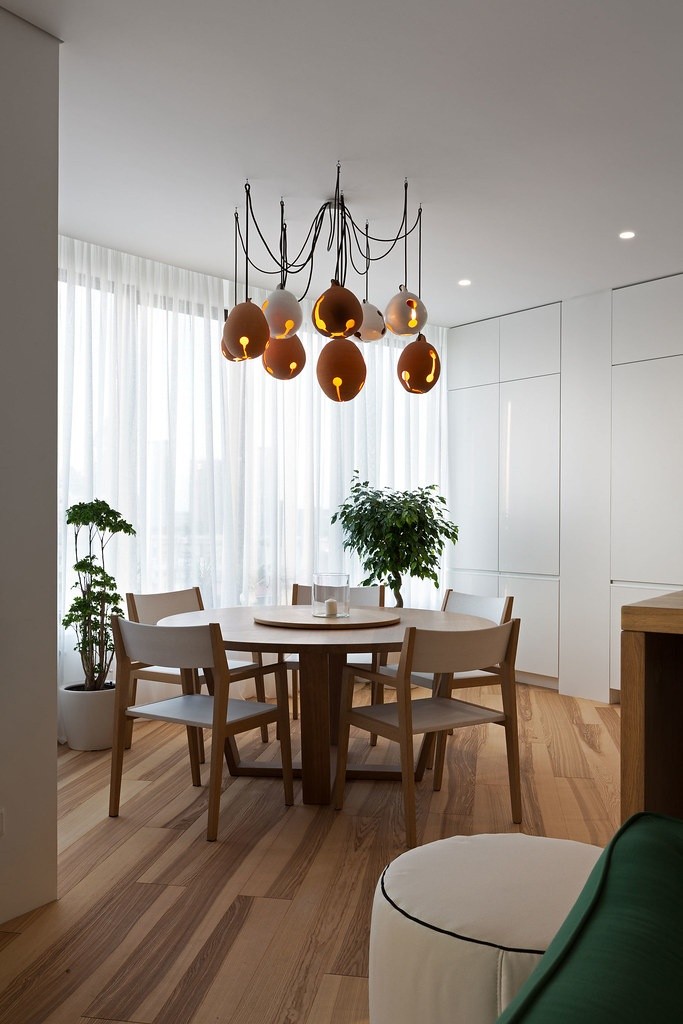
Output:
<box><xmin>331</xmin><ymin>617</ymin><xmax>525</xmax><ymax>824</ymax></box>
<box><xmin>274</xmin><ymin>582</ymin><xmax>390</xmax><ymax>749</ymax></box>
<box><xmin>106</xmin><ymin>613</ymin><xmax>293</xmax><ymax>843</ymax></box>
<box><xmin>369</xmin><ymin>588</ymin><xmax>512</xmax><ymax>770</ymax></box>
<box><xmin>122</xmin><ymin>587</ymin><xmax>267</xmax><ymax>786</ymax></box>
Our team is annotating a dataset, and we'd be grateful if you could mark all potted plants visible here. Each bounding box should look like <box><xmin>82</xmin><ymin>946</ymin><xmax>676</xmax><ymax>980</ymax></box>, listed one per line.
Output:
<box><xmin>329</xmin><ymin>470</ymin><xmax>460</xmax><ymax>690</ymax></box>
<box><xmin>58</xmin><ymin>497</ymin><xmax>138</xmax><ymax>752</ymax></box>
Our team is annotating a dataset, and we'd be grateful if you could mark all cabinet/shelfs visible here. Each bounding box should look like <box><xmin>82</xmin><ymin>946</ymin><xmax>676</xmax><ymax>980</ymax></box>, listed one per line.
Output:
<box><xmin>442</xmin><ymin>270</ymin><xmax>683</xmax><ymax>704</ymax></box>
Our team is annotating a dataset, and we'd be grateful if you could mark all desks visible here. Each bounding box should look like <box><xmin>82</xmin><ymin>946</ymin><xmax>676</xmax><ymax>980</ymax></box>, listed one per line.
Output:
<box><xmin>616</xmin><ymin>587</ymin><xmax>683</xmax><ymax>830</ymax></box>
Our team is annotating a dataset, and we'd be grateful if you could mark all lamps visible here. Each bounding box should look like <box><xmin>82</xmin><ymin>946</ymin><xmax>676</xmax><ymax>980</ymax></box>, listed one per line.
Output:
<box><xmin>218</xmin><ymin>162</ymin><xmax>441</xmax><ymax>403</ymax></box>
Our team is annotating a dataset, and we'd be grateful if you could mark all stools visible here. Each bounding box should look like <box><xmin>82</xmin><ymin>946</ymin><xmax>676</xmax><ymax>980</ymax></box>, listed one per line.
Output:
<box><xmin>368</xmin><ymin>830</ymin><xmax>605</xmax><ymax>1023</ymax></box>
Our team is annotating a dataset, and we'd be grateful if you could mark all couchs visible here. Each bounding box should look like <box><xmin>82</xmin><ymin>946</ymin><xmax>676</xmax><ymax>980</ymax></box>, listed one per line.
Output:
<box><xmin>492</xmin><ymin>812</ymin><xmax>682</xmax><ymax>1024</ymax></box>
